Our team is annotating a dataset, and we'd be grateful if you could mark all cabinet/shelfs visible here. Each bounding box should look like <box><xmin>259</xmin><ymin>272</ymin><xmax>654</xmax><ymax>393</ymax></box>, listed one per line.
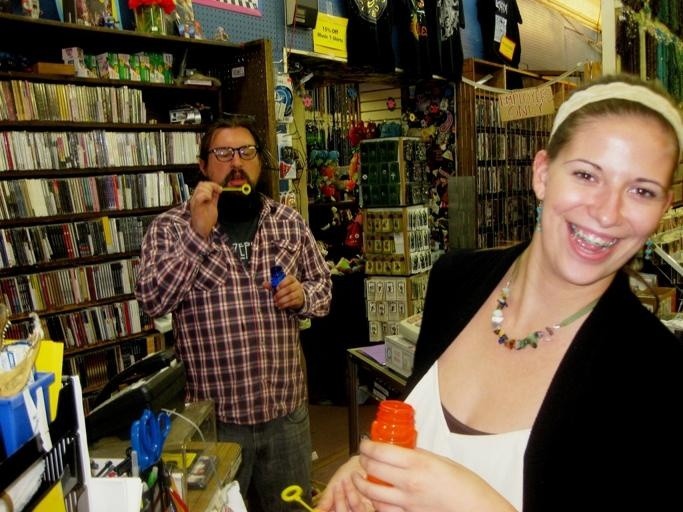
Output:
<box><xmin>455</xmin><ymin>57</ymin><xmax>581</xmax><ymax>254</ymax></box>
<box><xmin>0</xmin><ymin>15</ymin><xmax>280</xmax><ymax>418</ymax></box>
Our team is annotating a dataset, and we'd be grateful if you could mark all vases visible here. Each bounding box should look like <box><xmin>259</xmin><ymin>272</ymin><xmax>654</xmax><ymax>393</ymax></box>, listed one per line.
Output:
<box><xmin>133</xmin><ymin>7</ymin><xmax>163</xmax><ymax>33</ymax></box>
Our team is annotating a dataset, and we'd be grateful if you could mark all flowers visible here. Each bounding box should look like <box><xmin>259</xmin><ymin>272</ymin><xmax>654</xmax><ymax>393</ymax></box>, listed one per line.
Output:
<box><xmin>127</xmin><ymin>0</ymin><xmax>177</xmax><ymax>31</ymax></box>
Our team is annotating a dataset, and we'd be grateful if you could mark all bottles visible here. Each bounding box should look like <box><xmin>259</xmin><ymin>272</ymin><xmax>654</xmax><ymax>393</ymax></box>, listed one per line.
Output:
<box><xmin>584</xmin><ymin>58</ymin><xmax>602</xmax><ymax>85</ymax></box>
<box><xmin>367</xmin><ymin>400</ymin><xmax>416</xmax><ymax>487</ymax></box>
<box><xmin>271</xmin><ymin>265</ymin><xmax>286</xmax><ymax>294</ymax></box>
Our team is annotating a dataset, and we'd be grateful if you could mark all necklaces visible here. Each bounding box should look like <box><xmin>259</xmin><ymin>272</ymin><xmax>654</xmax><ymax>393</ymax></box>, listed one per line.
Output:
<box><xmin>493</xmin><ymin>253</ymin><xmax>604</xmax><ymax>350</ymax></box>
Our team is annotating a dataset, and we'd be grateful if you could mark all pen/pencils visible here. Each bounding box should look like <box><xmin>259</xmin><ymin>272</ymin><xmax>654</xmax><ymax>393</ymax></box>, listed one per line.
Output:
<box><xmin>146</xmin><ymin>466</ymin><xmax>159</xmax><ymax>490</ymax></box>
<box><xmin>131</xmin><ymin>450</ymin><xmax>139</xmax><ymax>477</ymax></box>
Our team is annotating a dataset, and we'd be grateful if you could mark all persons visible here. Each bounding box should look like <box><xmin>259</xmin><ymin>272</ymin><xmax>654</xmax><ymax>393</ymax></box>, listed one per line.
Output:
<box><xmin>136</xmin><ymin>114</ymin><xmax>333</xmax><ymax>509</ymax></box>
<box><xmin>318</xmin><ymin>74</ymin><xmax>681</xmax><ymax>509</ymax></box>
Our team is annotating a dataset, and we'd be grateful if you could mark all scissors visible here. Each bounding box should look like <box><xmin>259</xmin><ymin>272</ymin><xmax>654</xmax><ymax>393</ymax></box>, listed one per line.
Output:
<box><xmin>131</xmin><ymin>409</ymin><xmax>171</xmax><ymax>473</ymax></box>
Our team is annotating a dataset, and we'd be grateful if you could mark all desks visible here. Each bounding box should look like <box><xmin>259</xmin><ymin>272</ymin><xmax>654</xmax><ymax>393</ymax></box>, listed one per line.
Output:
<box><xmin>346</xmin><ymin>341</ymin><xmax>411</xmax><ymax>457</ymax></box>
<box><xmin>33</xmin><ymin>434</ymin><xmax>242</xmax><ymax>512</ymax></box>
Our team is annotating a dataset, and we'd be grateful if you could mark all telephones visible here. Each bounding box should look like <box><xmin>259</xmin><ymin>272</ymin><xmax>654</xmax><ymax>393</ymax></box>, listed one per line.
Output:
<box><xmin>85</xmin><ymin>350</ymin><xmax>187</xmax><ymax>446</ymax></box>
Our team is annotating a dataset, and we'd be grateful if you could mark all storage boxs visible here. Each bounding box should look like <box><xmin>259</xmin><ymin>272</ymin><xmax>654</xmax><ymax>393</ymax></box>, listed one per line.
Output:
<box><xmin>384</xmin><ymin>334</ymin><xmax>417</xmax><ymax>379</ymax></box>
<box><xmin>636</xmin><ymin>284</ymin><xmax>678</xmax><ymax>323</ymax></box>
<box><xmin>0</xmin><ymin>368</ymin><xmax>54</xmax><ymax>451</ymax></box>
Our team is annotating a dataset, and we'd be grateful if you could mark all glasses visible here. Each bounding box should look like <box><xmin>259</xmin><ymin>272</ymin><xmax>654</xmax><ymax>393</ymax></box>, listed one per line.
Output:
<box><xmin>208</xmin><ymin>145</ymin><xmax>259</xmax><ymax>162</ymax></box>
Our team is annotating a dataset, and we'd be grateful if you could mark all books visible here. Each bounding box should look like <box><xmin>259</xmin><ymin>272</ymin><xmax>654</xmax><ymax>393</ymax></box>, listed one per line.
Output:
<box><xmin>0</xmin><ymin>80</ymin><xmax>205</xmax><ymax>408</ymax></box>
<box><xmin>162</xmin><ymin>450</ymin><xmax>219</xmax><ymax>489</ymax></box>
<box><xmin>475</xmin><ymin>90</ymin><xmax>556</xmax><ymax>248</ymax></box>
